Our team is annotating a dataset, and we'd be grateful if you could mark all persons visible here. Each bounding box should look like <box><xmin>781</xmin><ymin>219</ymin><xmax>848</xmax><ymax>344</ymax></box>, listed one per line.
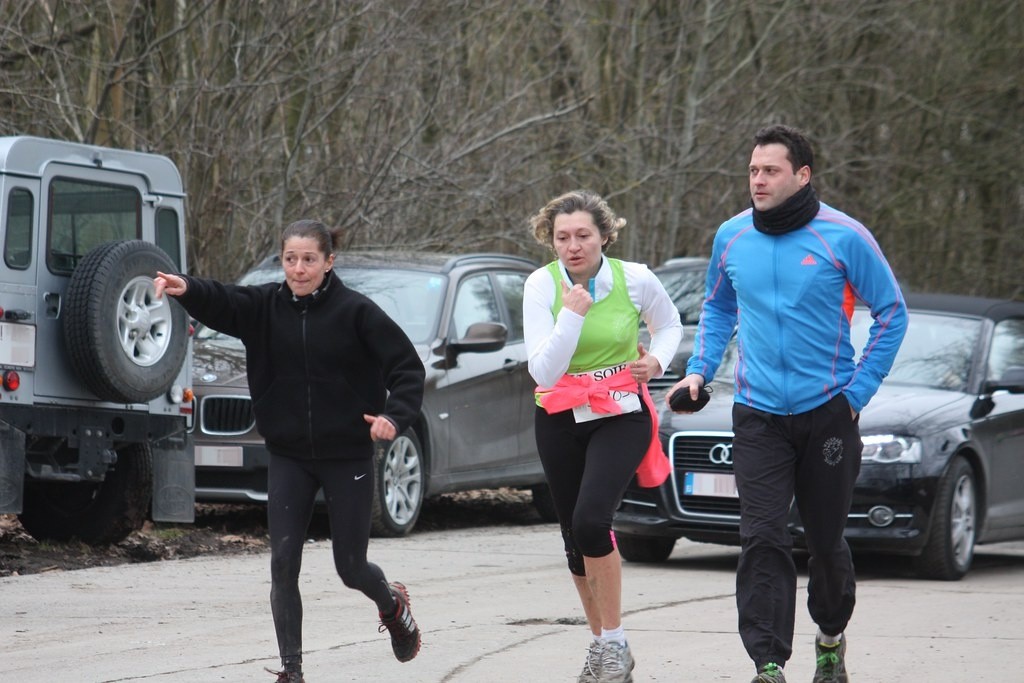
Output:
<box><xmin>154</xmin><ymin>219</ymin><xmax>426</xmax><ymax>683</ymax></box>
<box><xmin>522</xmin><ymin>190</ymin><xmax>683</xmax><ymax>683</ymax></box>
<box><xmin>666</xmin><ymin>125</ymin><xmax>909</xmax><ymax>683</ymax></box>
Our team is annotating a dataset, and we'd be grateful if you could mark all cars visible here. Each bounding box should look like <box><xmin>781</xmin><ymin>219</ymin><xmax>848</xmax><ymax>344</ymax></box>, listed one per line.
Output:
<box><xmin>613</xmin><ymin>293</ymin><xmax>1024</xmax><ymax>582</ymax></box>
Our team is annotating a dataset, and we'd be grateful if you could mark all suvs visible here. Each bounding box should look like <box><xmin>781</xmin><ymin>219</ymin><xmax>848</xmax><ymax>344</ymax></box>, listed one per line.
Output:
<box><xmin>639</xmin><ymin>257</ymin><xmax>754</xmax><ymax>406</ymax></box>
<box><xmin>185</xmin><ymin>250</ymin><xmax>562</xmax><ymax>541</ymax></box>
<box><xmin>1</xmin><ymin>133</ymin><xmax>197</xmax><ymax>548</ymax></box>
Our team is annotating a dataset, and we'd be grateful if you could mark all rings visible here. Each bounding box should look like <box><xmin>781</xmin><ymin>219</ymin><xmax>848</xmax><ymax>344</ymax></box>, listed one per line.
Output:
<box><xmin>636</xmin><ymin>374</ymin><xmax>639</xmax><ymax>380</ymax></box>
<box><xmin>635</xmin><ymin>362</ymin><xmax>637</xmax><ymax>368</ymax></box>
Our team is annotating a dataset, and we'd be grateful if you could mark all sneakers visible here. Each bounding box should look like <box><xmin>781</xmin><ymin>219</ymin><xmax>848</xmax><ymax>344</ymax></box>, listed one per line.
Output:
<box><xmin>812</xmin><ymin>632</ymin><xmax>847</xmax><ymax>683</ymax></box>
<box><xmin>751</xmin><ymin>662</ymin><xmax>786</xmax><ymax>683</ymax></box>
<box><xmin>378</xmin><ymin>581</ymin><xmax>422</xmax><ymax>663</ymax></box>
<box><xmin>598</xmin><ymin>639</ymin><xmax>635</xmax><ymax>683</ymax></box>
<box><xmin>263</xmin><ymin>667</ymin><xmax>306</xmax><ymax>683</ymax></box>
<box><xmin>578</xmin><ymin>640</ymin><xmax>602</xmax><ymax>683</ymax></box>
